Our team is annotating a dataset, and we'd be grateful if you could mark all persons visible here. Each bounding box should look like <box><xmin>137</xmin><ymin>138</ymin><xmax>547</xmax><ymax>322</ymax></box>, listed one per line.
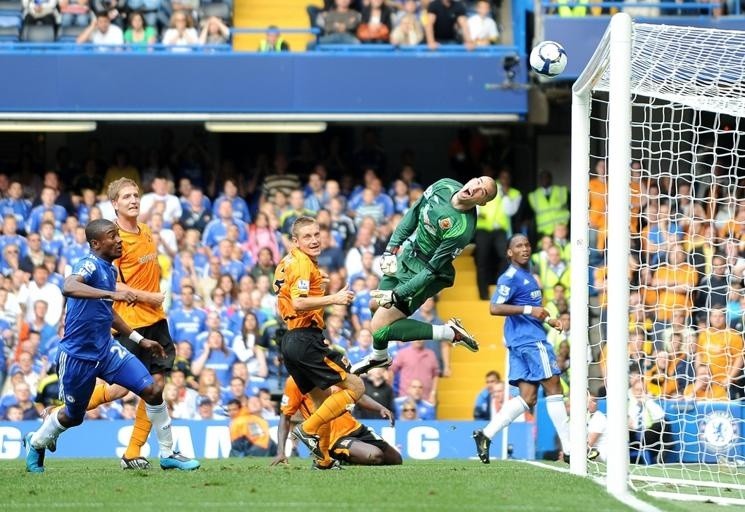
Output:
<box><xmin>1</xmin><ymin>158</ymin><xmax>745</xmax><ymax>471</ymax></box>
<box><xmin>16</xmin><ymin>1</ymin><xmax>745</xmax><ymax>50</ymax></box>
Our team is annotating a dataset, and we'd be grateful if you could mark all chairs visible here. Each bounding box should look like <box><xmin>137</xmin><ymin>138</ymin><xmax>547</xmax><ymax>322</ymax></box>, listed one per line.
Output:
<box><xmin>0</xmin><ymin>0</ymin><xmax>231</xmax><ymax>47</ymax></box>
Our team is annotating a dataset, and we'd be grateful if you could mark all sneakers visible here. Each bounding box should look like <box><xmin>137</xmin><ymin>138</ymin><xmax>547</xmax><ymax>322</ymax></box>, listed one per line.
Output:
<box><xmin>310</xmin><ymin>460</ymin><xmax>345</xmax><ymax>471</ymax></box>
<box><xmin>119</xmin><ymin>454</ymin><xmax>153</xmax><ymax>471</ymax></box>
<box><xmin>291</xmin><ymin>423</ymin><xmax>324</xmax><ymax>457</ymax></box>
<box><xmin>473</xmin><ymin>428</ymin><xmax>492</xmax><ymax>464</ymax></box>
<box><xmin>158</xmin><ymin>450</ymin><xmax>201</xmax><ymax>471</ymax></box>
<box><xmin>448</xmin><ymin>316</ymin><xmax>479</xmax><ymax>353</ymax></box>
<box><xmin>563</xmin><ymin>450</ymin><xmax>601</xmax><ymax>464</ymax></box>
<box><xmin>348</xmin><ymin>353</ymin><xmax>393</xmax><ymax>377</ymax></box>
<box><xmin>40</xmin><ymin>406</ymin><xmax>58</xmax><ymax>453</ymax></box>
<box><xmin>22</xmin><ymin>432</ymin><xmax>46</xmax><ymax>473</ymax></box>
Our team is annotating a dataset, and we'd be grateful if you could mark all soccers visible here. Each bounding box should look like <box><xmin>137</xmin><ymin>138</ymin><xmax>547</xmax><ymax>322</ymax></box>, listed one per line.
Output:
<box><xmin>528</xmin><ymin>39</ymin><xmax>570</xmax><ymax>78</ymax></box>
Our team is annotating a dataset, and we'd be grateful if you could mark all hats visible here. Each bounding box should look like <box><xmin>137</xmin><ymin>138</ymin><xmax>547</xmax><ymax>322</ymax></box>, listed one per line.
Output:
<box><xmin>194</xmin><ymin>394</ymin><xmax>213</xmax><ymax>408</ymax></box>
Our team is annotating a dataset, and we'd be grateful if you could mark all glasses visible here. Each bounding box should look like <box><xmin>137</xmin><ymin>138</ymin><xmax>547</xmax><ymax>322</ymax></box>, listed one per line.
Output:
<box><xmin>403</xmin><ymin>408</ymin><xmax>416</xmax><ymax>413</ymax></box>
